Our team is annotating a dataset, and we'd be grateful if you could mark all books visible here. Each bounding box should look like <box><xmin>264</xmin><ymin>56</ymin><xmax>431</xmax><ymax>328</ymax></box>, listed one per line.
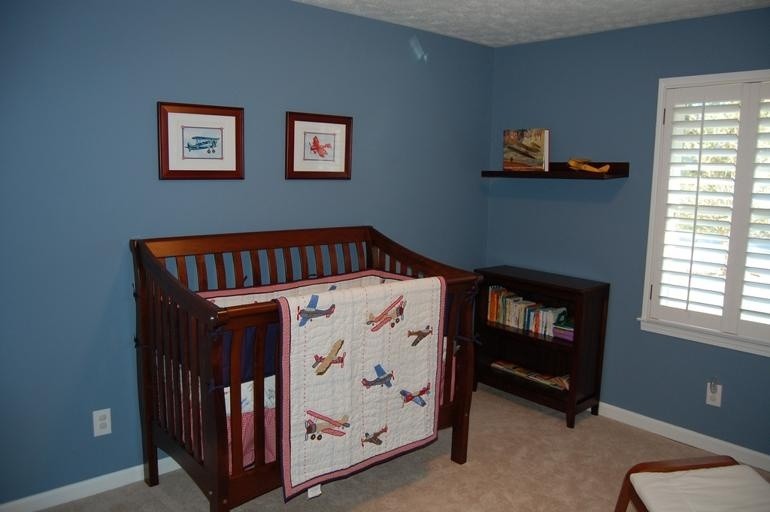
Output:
<box><xmin>492</xmin><ymin>358</ymin><xmax>571</xmax><ymax>392</ymax></box>
<box><xmin>502</xmin><ymin>127</ymin><xmax>550</xmax><ymax>172</ymax></box>
<box><xmin>487</xmin><ymin>284</ymin><xmax>575</xmax><ymax>342</ymax></box>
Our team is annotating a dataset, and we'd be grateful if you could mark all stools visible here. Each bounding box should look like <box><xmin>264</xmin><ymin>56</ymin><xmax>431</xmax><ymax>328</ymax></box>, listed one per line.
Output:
<box><xmin>616</xmin><ymin>454</ymin><xmax>769</xmax><ymax>512</ymax></box>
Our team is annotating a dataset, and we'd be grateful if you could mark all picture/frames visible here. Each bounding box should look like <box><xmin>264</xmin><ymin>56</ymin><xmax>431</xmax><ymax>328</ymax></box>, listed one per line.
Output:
<box><xmin>285</xmin><ymin>113</ymin><xmax>352</xmax><ymax>181</ymax></box>
<box><xmin>156</xmin><ymin>99</ymin><xmax>247</xmax><ymax>183</ymax></box>
<box><xmin>502</xmin><ymin>128</ymin><xmax>547</xmax><ymax>171</ymax></box>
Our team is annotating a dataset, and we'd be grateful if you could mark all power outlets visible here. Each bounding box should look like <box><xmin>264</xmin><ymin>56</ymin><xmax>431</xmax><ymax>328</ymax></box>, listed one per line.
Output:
<box><xmin>92</xmin><ymin>408</ymin><xmax>112</xmax><ymax>437</ymax></box>
<box><xmin>704</xmin><ymin>384</ymin><xmax>723</xmax><ymax>406</ymax></box>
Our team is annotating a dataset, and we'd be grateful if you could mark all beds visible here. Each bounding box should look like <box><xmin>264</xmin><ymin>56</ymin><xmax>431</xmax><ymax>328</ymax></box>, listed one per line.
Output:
<box><xmin>130</xmin><ymin>223</ymin><xmax>472</xmax><ymax>511</ymax></box>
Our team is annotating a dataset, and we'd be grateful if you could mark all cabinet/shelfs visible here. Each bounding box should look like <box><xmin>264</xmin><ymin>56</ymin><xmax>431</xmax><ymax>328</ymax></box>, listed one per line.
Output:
<box><xmin>474</xmin><ymin>263</ymin><xmax>610</xmax><ymax>427</ymax></box>
